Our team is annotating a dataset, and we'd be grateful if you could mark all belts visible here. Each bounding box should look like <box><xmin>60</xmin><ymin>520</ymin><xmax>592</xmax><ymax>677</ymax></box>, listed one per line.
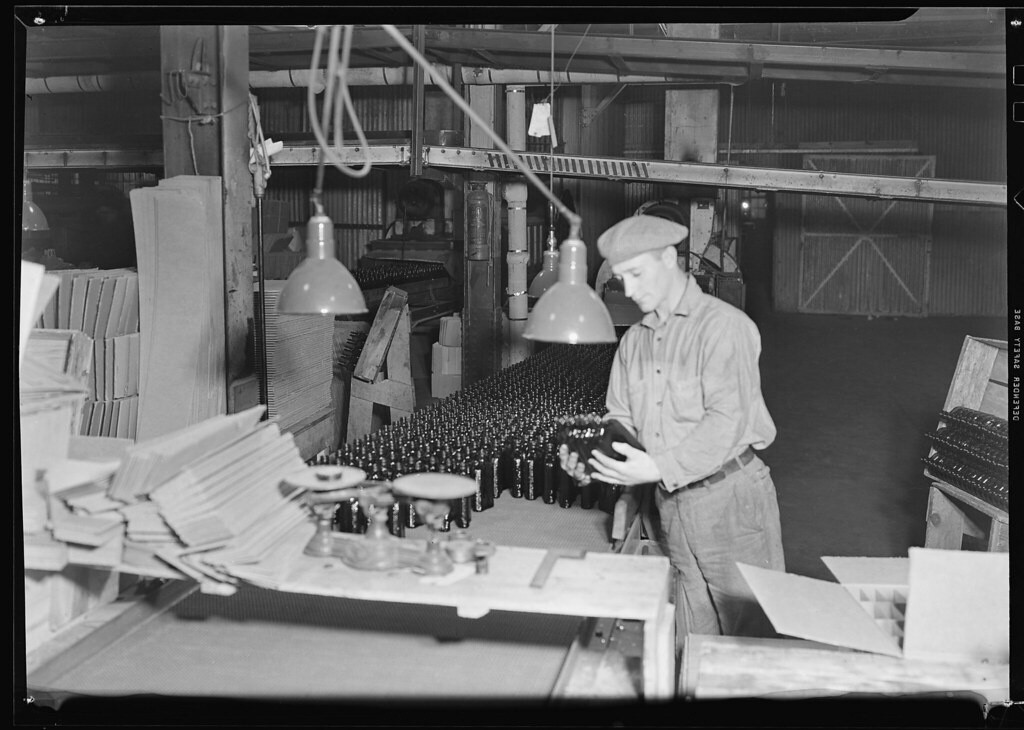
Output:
<box><xmin>666</xmin><ymin>446</ymin><xmax>755</xmax><ymax>495</ymax></box>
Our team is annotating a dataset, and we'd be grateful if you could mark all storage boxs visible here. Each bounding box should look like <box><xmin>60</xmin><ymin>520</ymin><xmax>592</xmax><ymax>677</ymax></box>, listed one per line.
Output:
<box><xmin>739</xmin><ymin>547</ymin><xmax>1010</xmax><ymax>666</ymax></box>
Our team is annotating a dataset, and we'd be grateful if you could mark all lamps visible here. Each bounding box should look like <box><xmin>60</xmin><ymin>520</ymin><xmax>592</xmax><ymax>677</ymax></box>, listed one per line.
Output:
<box><xmin>22</xmin><ymin>178</ymin><xmax>49</xmax><ymax>231</ymax></box>
<box><xmin>272</xmin><ymin>25</ymin><xmax>370</xmax><ymax>315</ymax></box>
<box><xmin>378</xmin><ymin>24</ymin><xmax>618</xmax><ymax>346</ymax></box>
<box><xmin>527</xmin><ymin>24</ymin><xmax>563</xmax><ymax>296</ymax></box>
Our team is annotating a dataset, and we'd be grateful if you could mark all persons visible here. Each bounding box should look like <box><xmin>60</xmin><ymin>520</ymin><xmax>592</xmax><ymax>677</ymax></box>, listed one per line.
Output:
<box><xmin>557</xmin><ymin>214</ymin><xmax>785</xmax><ymax>671</ymax></box>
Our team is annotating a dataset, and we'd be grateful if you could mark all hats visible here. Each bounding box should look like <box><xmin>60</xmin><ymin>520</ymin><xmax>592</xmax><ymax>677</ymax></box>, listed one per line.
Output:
<box><xmin>597</xmin><ymin>215</ymin><xmax>688</xmax><ymax>264</ymax></box>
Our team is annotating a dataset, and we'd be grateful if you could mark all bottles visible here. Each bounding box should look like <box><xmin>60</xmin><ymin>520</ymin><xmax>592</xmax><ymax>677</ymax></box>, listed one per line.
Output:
<box><xmin>308</xmin><ymin>325</ymin><xmax>645</xmax><ymax>537</ymax></box>
<box><xmin>919</xmin><ymin>406</ymin><xmax>1009</xmax><ymax>512</ymax></box>
<box><xmin>350</xmin><ymin>262</ymin><xmax>445</xmax><ymax>290</ymax></box>
